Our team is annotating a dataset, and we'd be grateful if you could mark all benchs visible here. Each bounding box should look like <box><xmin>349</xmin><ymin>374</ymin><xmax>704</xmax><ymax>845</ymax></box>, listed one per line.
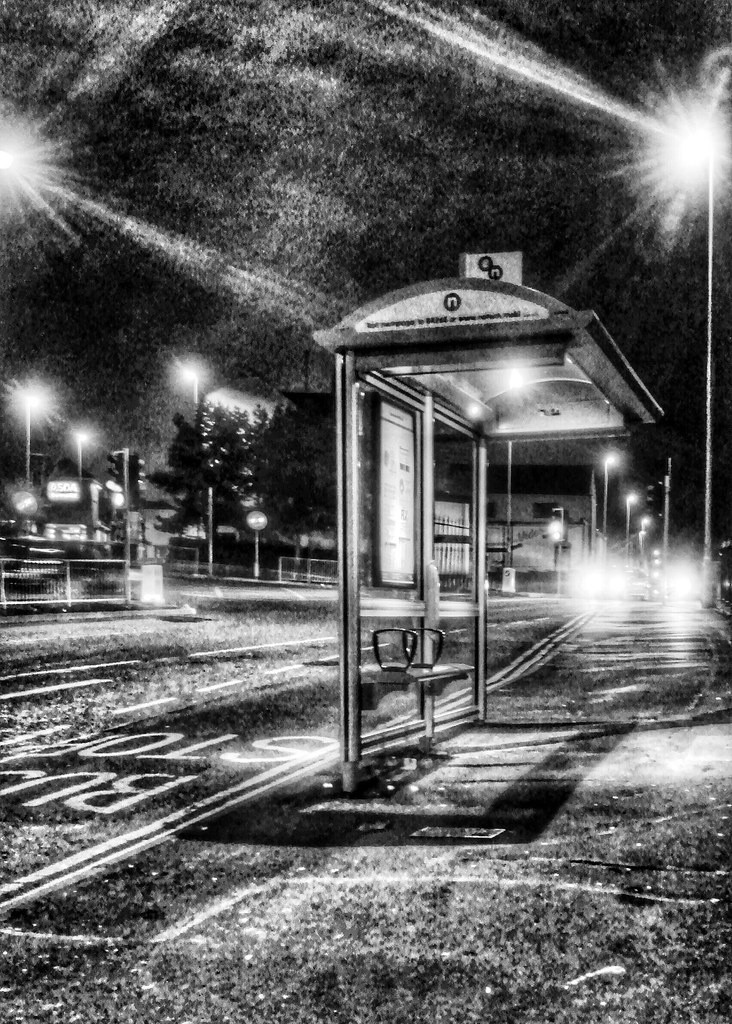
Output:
<box><xmin>356</xmin><ymin>627</ymin><xmax>477</xmax><ymax>711</ymax></box>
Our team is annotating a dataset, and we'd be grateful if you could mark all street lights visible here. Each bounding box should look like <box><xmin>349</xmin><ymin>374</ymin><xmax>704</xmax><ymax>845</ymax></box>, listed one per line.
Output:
<box><xmin>622</xmin><ymin>490</ymin><xmax>638</xmax><ymax>546</ymax></box>
<box><xmin>13</xmin><ymin>380</ymin><xmax>48</xmax><ymax>485</ymax></box>
<box><xmin>599</xmin><ymin>451</ymin><xmax>619</xmax><ymax>534</ymax></box>
<box><xmin>636</xmin><ymin>514</ymin><xmax>652</xmax><ymax>563</ymax></box>
<box><xmin>68</xmin><ymin>426</ymin><xmax>94</xmax><ymax>483</ymax></box>
<box><xmin>179</xmin><ymin>368</ymin><xmax>202</xmax><ymax>423</ymax></box>
